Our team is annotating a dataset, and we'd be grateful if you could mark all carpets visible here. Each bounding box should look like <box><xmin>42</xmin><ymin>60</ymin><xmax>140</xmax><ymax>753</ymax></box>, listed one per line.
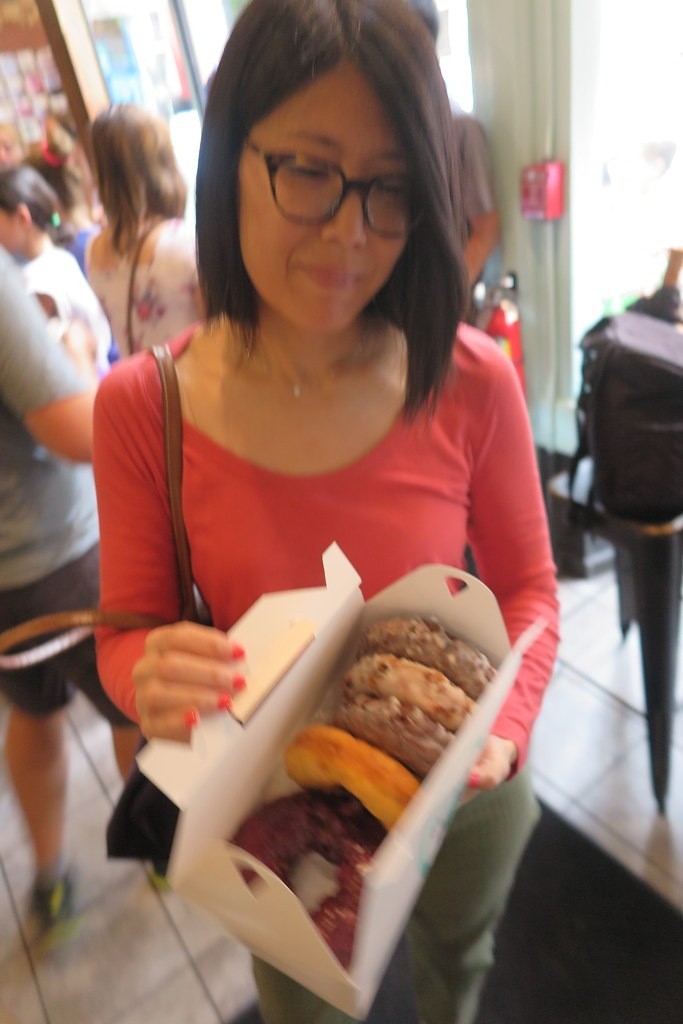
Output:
<box><xmin>230</xmin><ymin>801</ymin><xmax>683</xmax><ymax>1024</ymax></box>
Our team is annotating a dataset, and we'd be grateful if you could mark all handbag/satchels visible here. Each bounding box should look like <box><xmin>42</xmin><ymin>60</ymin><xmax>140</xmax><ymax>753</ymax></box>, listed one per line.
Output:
<box><xmin>0</xmin><ymin>343</ymin><xmax>215</xmax><ymax>877</ymax></box>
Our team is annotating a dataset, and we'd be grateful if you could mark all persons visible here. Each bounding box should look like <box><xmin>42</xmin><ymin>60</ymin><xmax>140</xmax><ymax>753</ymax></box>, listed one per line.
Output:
<box><xmin>0</xmin><ymin>106</ymin><xmax>209</xmax><ymax>957</ymax></box>
<box><xmin>91</xmin><ymin>0</ymin><xmax>563</xmax><ymax>1024</ymax></box>
<box><xmin>411</xmin><ymin>0</ymin><xmax>503</xmax><ymax>330</ymax></box>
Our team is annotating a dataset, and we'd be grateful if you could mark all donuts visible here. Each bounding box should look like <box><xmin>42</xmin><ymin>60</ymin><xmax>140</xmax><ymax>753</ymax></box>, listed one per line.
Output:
<box><xmin>225</xmin><ymin>614</ymin><xmax>501</xmax><ymax>976</ymax></box>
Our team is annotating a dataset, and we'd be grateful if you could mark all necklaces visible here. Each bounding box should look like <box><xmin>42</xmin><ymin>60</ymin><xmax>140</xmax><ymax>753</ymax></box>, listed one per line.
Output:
<box><xmin>248</xmin><ymin>347</ymin><xmax>365</xmax><ymax>398</ymax></box>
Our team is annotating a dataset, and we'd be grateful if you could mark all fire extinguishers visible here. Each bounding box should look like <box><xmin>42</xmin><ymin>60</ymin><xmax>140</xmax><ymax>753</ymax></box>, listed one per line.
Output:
<box><xmin>470</xmin><ymin>279</ymin><xmax>526</xmax><ymax>410</ymax></box>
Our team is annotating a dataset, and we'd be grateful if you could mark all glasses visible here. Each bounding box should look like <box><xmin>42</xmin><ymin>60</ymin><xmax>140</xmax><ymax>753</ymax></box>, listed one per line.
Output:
<box><xmin>246</xmin><ymin>137</ymin><xmax>429</xmax><ymax>235</ymax></box>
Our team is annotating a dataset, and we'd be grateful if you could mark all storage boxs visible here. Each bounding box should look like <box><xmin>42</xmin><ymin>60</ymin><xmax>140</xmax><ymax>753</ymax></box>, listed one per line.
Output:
<box><xmin>135</xmin><ymin>540</ymin><xmax>550</xmax><ymax>1022</ymax></box>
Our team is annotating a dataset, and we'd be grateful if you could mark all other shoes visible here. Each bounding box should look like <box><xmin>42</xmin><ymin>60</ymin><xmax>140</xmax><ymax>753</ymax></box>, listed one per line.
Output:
<box><xmin>27</xmin><ymin>869</ymin><xmax>78</xmax><ymax>954</ymax></box>
<box><xmin>144</xmin><ymin>861</ymin><xmax>170</xmax><ymax>893</ymax></box>
